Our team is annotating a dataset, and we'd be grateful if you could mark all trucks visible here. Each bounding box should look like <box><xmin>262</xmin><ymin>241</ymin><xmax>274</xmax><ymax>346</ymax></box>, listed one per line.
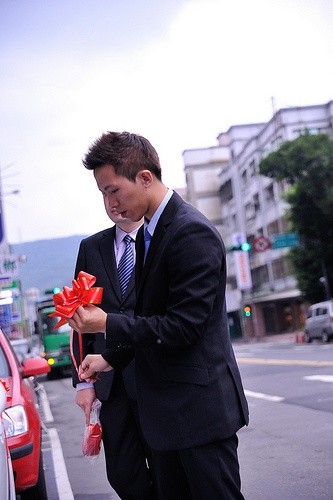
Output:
<box><xmin>34</xmin><ymin>293</ymin><xmax>75</xmax><ymax>381</ymax></box>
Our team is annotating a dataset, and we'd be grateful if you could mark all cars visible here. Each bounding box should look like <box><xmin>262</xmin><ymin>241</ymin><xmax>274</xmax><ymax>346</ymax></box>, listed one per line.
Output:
<box><xmin>0</xmin><ymin>381</ymin><xmax>16</xmax><ymax>500</ymax></box>
<box><xmin>10</xmin><ymin>333</ymin><xmax>43</xmax><ymax>365</ymax></box>
<box><xmin>0</xmin><ymin>328</ymin><xmax>51</xmax><ymax>500</ymax></box>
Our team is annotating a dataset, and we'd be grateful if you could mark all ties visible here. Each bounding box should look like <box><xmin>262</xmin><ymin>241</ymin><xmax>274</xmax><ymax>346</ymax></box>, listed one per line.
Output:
<box><xmin>142</xmin><ymin>227</ymin><xmax>151</xmax><ymax>266</ymax></box>
<box><xmin>117</xmin><ymin>236</ymin><xmax>135</xmax><ymax>298</ymax></box>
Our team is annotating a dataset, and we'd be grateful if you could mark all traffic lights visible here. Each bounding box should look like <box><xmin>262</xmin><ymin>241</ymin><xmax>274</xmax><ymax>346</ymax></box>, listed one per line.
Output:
<box><xmin>45</xmin><ymin>287</ymin><xmax>61</xmax><ymax>293</ymax></box>
<box><xmin>243</xmin><ymin>306</ymin><xmax>251</xmax><ymax>317</ymax></box>
<box><xmin>229</xmin><ymin>242</ymin><xmax>253</xmax><ymax>252</ymax></box>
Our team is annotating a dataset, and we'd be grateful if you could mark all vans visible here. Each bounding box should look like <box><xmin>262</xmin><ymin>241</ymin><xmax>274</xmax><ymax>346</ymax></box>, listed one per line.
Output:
<box><xmin>304</xmin><ymin>300</ymin><xmax>333</xmax><ymax>343</ymax></box>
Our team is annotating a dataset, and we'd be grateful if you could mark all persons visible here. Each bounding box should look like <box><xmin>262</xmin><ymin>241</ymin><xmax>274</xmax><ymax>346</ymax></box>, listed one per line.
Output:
<box><xmin>70</xmin><ymin>193</ymin><xmax>145</xmax><ymax>427</ymax></box>
<box><xmin>66</xmin><ymin>131</ymin><xmax>249</xmax><ymax>500</ymax></box>
